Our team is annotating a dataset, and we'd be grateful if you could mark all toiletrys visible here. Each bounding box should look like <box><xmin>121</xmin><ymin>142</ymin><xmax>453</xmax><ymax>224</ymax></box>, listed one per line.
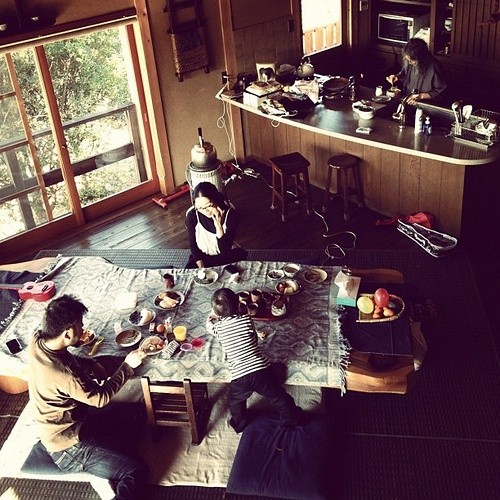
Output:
<box><xmin>423</xmin><ymin>116</ymin><xmax>432</xmax><ymax>134</ymax></box>
<box><xmin>414</xmin><ymin>107</ymin><xmax>424</xmax><ymax>131</ymax></box>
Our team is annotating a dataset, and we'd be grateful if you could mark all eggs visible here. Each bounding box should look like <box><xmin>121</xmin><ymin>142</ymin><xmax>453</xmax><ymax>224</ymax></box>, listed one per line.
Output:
<box><xmin>156</xmin><ymin>323</ymin><xmax>165</xmax><ymax>333</ymax></box>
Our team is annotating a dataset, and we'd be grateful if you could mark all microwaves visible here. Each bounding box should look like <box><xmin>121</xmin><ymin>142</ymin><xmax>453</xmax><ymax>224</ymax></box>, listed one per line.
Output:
<box><xmin>378</xmin><ymin>10</ymin><xmax>430</xmax><ymax>43</ymax></box>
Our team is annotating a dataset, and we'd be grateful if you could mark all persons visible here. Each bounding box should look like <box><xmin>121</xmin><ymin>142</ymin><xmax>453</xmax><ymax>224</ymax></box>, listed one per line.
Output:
<box><xmin>206</xmin><ymin>288</ymin><xmax>304</xmax><ymax>435</ymax></box>
<box><xmin>183</xmin><ymin>182</ymin><xmax>249</xmax><ymax>269</ymax></box>
<box><xmin>386</xmin><ymin>38</ymin><xmax>449</xmax><ymax>107</ymax></box>
<box><xmin>26</xmin><ymin>293</ymin><xmax>152</xmax><ymax>500</ymax></box>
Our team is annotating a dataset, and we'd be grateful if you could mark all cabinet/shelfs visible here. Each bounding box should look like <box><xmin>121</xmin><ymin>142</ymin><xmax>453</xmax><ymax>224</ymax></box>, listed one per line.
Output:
<box><xmin>370</xmin><ymin>0</ymin><xmax>500</xmax><ymax>110</ymax></box>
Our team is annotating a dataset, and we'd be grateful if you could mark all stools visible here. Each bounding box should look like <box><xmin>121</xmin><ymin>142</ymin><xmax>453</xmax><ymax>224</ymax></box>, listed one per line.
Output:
<box><xmin>268</xmin><ymin>152</ymin><xmax>314</xmax><ymax>223</ymax></box>
<box><xmin>321</xmin><ymin>154</ymin><xmax>366</xmax><ymax>224</ymax></box>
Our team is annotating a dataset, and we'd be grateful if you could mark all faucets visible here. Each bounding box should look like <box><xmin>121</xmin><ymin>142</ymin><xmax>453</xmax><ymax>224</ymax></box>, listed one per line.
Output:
<box><xmin>393</xmin><ymin>92</ymin><xmax>413</xmax><ymax>128</ymax></box>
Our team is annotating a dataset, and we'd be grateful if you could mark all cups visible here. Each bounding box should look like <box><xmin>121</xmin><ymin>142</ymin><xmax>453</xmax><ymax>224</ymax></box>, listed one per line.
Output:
<box><xmin>238</xmin><ymin>301</ymin><xmax>247</xmax><ymax>314</ymax></box>
<box><xmin>173</xmin><ymin>326</ymin><xmax>186</xmax><ymax>342</ymax></box>
<box><xmin>271</xmin><ymin>301</ymin><xmax>286</xmax><ymax>316</ymax></box>
<box><xmin>238</xmin><ymin>290</ymin><xmax>249</xmax><ymax>302</ymax></box>
<box><xmin>445</xmin><ymin>17</ymin><xmax>452</xmax><ymax>31</ymax></box>
<box><xmin>164</xmin><ymin>273</ymin><xmax>174</xmax><ymax>289</ymax></box>
<box><xmin>247</xmin><ymin>302</ymin><xmax>259</xmax><ymax>316</ymax></box>
<box><xmin>262</xmin><ymin>292</ymin><xmax>273</xmax><ymax>304</ymax></box>
<box><xmin>251</xmin><ymin>288</ymin><xmax>262</xmax><ymax>303</ymax></box>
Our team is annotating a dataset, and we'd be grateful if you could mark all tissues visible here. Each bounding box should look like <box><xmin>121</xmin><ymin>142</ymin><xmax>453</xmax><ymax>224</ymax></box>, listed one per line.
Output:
<box><xmin>333</xmin><ymin>270</ymin><xmax>362</xmax><ymax>307</ymax></box>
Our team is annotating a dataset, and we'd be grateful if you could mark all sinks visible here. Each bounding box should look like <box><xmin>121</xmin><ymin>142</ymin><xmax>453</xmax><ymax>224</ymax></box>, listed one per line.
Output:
<box><xmin>373</xmin><ymin>99</ymin><xmax>458</xmax><ymax>135</ymax></box>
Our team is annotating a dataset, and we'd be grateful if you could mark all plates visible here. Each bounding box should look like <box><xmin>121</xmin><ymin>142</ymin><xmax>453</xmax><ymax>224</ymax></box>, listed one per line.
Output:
<box><xmin>116</xmin><ymin>329</ymin><xmax>141</xmax><ymax>348</ymax></box>
<box><xmin>267</xmin><ymin>270</ymin><xmax>285</xmax><ymax>280</ymax></box>
<box><xmin>128</xmin><ymin>308</ymin><xmax>157</xmax><ymax>327</ymax></box>
<box><xmin>372</xmin><ymin>95</ymin><xmax>391</xmax><ymax>103</ymax></box>
<box><xmin>193</xmin><ymin>269</ymin><xmax>219</xmax><ymax>285</ymax></box>
<box><xmin>139</xmin><ymin>334</ymin><xmax>168</xmax><ymax>355</ymax></box>
<box><xmin>153</xmin><ymin>290</ymin><xmax>185</xmax><ymax>311</ymax></box>
<box><xmin>275</xmin><ymin>278</ymin><xmax>302</xmax><ymax>295</ymax></box>
<box><xmin>77</xmin><ymin>330</ymin><xmax>96</xmax><ymax>345</ymax></box>
<box><xmin>303</xmin><ymin>269</ymin><xmax>328</xmax><ymax>284</ymax></box>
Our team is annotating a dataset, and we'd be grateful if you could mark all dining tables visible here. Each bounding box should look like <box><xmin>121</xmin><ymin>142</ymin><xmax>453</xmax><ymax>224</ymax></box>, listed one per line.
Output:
<box><xmin>0</xmin><ymin>255</ymin><xmax>411</xmax><ymax>395</ymax></box>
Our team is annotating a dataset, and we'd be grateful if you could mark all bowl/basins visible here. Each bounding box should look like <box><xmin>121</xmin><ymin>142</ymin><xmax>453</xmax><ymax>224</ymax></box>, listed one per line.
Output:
<box><xmin>180</xmin><ymin>338</ymin><xmax>205</xmax><ymax>351</ymax></box>
<box><xmin>283</xmin><ymin>264</ymin><xmax>300</xmax><ymax>278</ymax></box>
<box><xmin>386</xmin><ymin>89</ymin><xmax>401</xmax><ymax>98</ymax></box>
<box><xmin>359</xmin><ymin>106</ymin><xmax>376</xmax><ymax>119</ymax></box>
<box><xmin>352</xmin><ymin>101</ymin><xmax>364</xmax><ymax>112</ymax></box>
<box><xmin>323</xmin><ymin>77</ymin><xmax>349</xmax><ymax>92</ymax></box>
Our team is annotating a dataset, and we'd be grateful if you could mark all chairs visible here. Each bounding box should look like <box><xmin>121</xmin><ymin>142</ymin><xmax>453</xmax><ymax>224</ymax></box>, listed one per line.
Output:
<box><xmin>140</xmin><ymin>376</ymin><xmax>209</xmax><ymax>446</ymax></box>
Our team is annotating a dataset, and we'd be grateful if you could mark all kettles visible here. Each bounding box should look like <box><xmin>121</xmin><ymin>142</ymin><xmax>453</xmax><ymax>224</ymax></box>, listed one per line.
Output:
<box><xmin>297</xmin><ymin>55</ymin><xmax>315</xmax><ymax>78</ymax></box>
<box><xmin>191</xmin><ymin>128</ymin><xmax>217</xmax><ymax>168</ymax></box>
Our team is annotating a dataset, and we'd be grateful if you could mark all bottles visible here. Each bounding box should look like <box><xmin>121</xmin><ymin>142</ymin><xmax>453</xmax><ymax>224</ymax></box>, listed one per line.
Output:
<box><xmin>399</xmin><ymin>101</ymin><xmax>406</xmax><ymax>129</ymax></box>
<box><xmin>415</xmin><ymin>107</ymin><xmax>424</xmax><ymax>133</ymax></box>
<box><xmin>423</xmin><ymin>116</ymin><xmax>432</xmax><ymax>135</ymax></box>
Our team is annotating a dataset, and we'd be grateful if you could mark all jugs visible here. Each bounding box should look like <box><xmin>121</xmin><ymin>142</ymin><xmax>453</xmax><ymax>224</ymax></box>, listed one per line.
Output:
<box><xmin>375</xmin><ymin>86</ymin><xmax>383</xmax><ymax>96</ymax></box>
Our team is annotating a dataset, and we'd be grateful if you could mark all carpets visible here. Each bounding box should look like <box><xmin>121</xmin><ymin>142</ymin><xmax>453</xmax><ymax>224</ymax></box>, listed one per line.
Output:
<box><xmin>0</xmin><ymin>386</ymin><xmax>328</xmax><ymax>489</ymax></box>
<box><xmin>0</xmin><ymin>249</ymin><xmax>500</xmax><ymax>499</ymax></box>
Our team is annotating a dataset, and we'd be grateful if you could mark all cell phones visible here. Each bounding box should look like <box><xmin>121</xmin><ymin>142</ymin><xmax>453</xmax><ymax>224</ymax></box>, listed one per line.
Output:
<box><xmin>244</xmin><ymin>167</ymin><xmax>261</xmax><ymax>179</ymax></box>
<box><xmin>5</xmin><ymin>338</ymin><xmax>23</xmax><ymax>355</ymax></box>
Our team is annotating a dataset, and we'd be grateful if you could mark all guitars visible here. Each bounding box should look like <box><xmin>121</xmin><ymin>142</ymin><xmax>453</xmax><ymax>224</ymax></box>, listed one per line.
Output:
<box><xmin>0</xmin><ymin>280</ymin><xmax>56</xmax><ymax>302</ymax></box>
